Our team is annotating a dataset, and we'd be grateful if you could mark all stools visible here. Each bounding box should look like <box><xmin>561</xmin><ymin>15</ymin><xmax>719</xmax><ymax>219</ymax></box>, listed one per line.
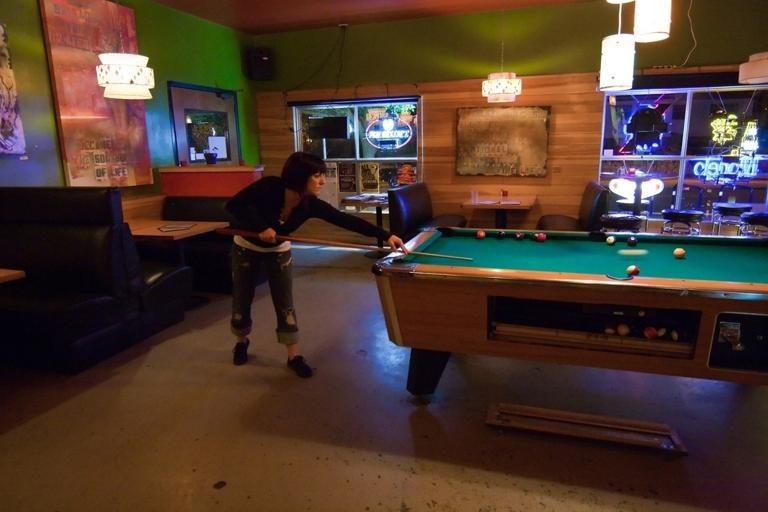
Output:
<box><xmin>601</xmin><ymin>200</ymin><xmax>768</xmax><ymax>237</ymax></box>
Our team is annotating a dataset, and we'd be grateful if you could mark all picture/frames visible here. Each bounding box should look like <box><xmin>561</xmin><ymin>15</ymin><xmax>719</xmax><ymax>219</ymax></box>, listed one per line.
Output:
<box><xmin>167</xmin><ymin>81</ymin><xmax>242</xmax><ymax>166</ymax></box>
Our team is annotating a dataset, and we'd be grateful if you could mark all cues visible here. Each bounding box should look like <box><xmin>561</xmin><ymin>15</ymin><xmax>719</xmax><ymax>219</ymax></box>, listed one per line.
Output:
<box><xmin>213</xmin><ymin>227</ymin><xmax>473</xmax><ymax>262</ymax></box>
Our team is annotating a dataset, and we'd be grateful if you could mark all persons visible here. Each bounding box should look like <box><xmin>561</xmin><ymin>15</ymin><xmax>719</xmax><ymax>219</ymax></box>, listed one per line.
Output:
<box><xmin>223</xmin><ymin>152</ymin><xmax>409</xmax><ymax>378</ymax></box>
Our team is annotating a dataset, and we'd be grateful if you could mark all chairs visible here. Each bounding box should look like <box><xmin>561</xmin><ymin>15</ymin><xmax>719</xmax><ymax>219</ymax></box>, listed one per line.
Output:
<box><xmin>387</xmin><ymin>182</ymin><xmax>467</xmax><ymax>244</ymax></box>
<box><xmin>537</xmin><ymin>180</ymin><xmax>609</xmax><ymax>231</ymax></box>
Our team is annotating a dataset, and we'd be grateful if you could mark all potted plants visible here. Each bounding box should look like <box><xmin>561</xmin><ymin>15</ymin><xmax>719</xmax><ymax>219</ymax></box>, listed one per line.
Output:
<box><xmin>202</xmin><ymin>144</ymin><xmax>218</xmax><ymax>164</ymax></box>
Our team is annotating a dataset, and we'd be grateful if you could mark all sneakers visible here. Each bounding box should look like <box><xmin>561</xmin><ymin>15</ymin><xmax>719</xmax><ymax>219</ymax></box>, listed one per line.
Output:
<box><xmin>287</xmin><ymin>356</ymin><xmax>313</xmax><ymax>378</ymax></box>
<box><xmin>232</xmin><ymin>338</ymin><xmax>250</xmax><ymax>366</ymax></box>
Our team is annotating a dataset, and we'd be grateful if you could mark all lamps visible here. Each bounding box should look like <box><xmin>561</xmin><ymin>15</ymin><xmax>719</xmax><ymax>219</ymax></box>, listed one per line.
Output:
<box><xmin>738</xmin><ymin>52</ymin><xmax>768</xmax><ymax>85</ymax></box>
<box><xmin>481</xmin><ymin>41</ymin><xmax>522</xmax><ymax>103</ymax></box>
<box><xmin>600</xmin><ymin>1</ymin><xmax>635</xmax><ymax>91</ymax></box>
<box><xmin>634</xmin><ymin>1</ymin><xmax>671</xmax><ymax>43</ymax></box>
<box><xmin>96</xmin><ymin>2</ymin><xmax>155</xmax><ymax>99</ymax></box>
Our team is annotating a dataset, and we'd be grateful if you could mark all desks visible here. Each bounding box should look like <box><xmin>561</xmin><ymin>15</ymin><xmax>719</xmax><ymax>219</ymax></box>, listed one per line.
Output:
<box><xmin>461</xmin><ymin>196</ymin><xmax>536</xmax><ymax>229</ymax></box>
<box><xmin>0</xmin><ymin>269</ymin><xmax>26</xmax><ymax>284</ymax></box>
<box><xmin>372</xmin><ymin>224</ymin><xmax>768</xmax><ymax>395</ymax></box>
<box><xmin>130</xmin><ymin>220</ymin><xmax>230</xmax><ymax>311</ymax></box>
<box><xmin>340</xmin><ymin>193</ymin><xmax>391</xmax><ymax>259</ymax></box>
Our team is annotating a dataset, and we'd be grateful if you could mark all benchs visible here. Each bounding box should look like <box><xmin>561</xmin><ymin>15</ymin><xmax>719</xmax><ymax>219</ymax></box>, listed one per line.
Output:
<box><xmin>122</xmin><ymin>223</ymin><xmax>192</xmax><ymax>339</ymax></box>
<box><xmin>0</xmin><ymin>221</ymin><xmax>127</xmax><ymax>380</ymax></box>
<box><xmin>161</xmin><ymin>196</ymin><xmax>267</xmax><ymax>293</ymax></box>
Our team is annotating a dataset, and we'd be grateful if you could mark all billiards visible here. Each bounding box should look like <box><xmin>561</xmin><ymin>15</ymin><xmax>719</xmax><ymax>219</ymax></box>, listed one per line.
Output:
<box><xmin>515</xmin><ymin>232</ymin><xmax>524</xmax><ymax>241</ymax></box>
<box><xmin>607</xmin><ymin>237</ymin><xmax>616</xmax><ymax>245</ymax></box>
<box><xmin>674</xmin><ymin>248</ymin><xmax>686</xmax><ymax>258</ymax></box>
<box><xmin>644</xmin><ymin>327</ymin><xmax>655</xmax><ymax>339</ymax></box>
<box><xmin>476</xmin><ymin>231</ymin><xmax>486</xmax><ymax>240</ymax></box>
<box><xmin>605</xmin><ymin>326</ymin><xmax>615</xmax><ymax>336</ymax></box>
<box><xmin>627</xmin><ymin>265</ymin><xmax>640</xmax><ymax>276</ymax></box>
<box><xmin>533</xmin><ymin>233</ymin><xmax>545</xmax><ymax>243</ymax></box>
<box><xmin>497</xmin><ymin>231</ymin><xmax>505</xmax><ymax>239</ymax></box>
<box><xmin>627</xmin><ymin>238</ymin><xmax>638</xmax><ymax>247</ymax></box>
<box><xmin>619</xmin><ymin>325</ymin><xmax>631</xmax><ymax>337</ymax></box>
